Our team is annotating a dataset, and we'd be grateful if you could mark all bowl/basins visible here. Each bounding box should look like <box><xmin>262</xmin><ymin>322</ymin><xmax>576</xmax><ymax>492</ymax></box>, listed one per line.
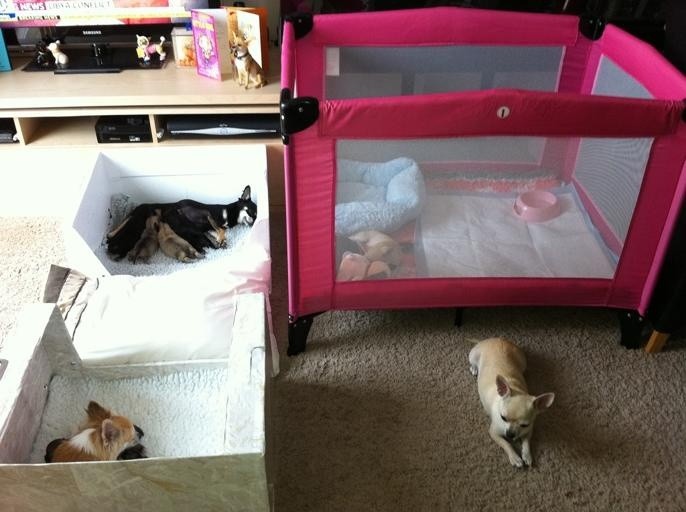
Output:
<box><xmin>511</xmin><ymin>190</ymin><xmax>561</xmax><ymax>222</ymax></box>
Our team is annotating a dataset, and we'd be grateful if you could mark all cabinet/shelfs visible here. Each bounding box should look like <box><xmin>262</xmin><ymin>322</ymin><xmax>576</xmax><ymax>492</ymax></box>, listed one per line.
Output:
<box><xmin>0</xmin><ymin>56</ymin><xmax>290</xmax><ymax>146</ymax></box>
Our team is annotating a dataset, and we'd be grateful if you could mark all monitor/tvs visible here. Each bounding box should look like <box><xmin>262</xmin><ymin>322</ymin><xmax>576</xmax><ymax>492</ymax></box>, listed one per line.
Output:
<box><xmin>0</xmin><ymin>0</ymin><xmax>221</xmax><ymax>74</ymax></box>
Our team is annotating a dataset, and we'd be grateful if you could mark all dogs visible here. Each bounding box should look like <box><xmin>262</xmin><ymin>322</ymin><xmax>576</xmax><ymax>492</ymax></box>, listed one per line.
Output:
<box><xmin>106</xmin><ymin>185</ymin><xmax>257</xmax><ymax>265</ymax></box>
<box><xmin>463</xmin><ymin>335</ymin><xmax>556</xmax><ymax>470</ymax></box>
<box><xmin>348</xmin><ymin>229</ymin><xmax>403</xmax><ymax>273</ymax></box>
<box><xmin>44</xmin><ymin>400</ymin><xmax>148</xmax><ymax>464</ymax></box>
